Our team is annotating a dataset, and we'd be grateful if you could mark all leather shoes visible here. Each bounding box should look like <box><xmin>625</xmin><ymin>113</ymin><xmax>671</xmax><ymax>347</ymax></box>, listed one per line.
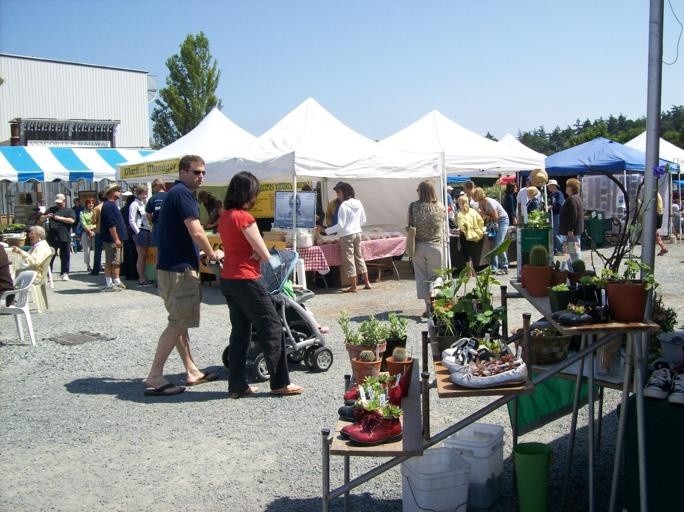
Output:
<box><xmin>337</xmin><ymin>374</ymin><xmax>410</xmax><ymax>445</ymax></box>
<box><xmin>441</xmin><ymin>337</ymin><xmax>529</xmax><ymax>389</ymax></box>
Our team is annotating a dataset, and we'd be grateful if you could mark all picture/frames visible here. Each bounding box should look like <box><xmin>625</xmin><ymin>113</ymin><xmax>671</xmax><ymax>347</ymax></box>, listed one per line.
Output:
<box><xmin>274</xmin><ymin>190</ymin><xmax>317</xmax><ymax>229</ymax></box>
<box><xmin>78</xmin><ymin>191</ymin><xmax>97</xmax><ymax>206</ymax></box>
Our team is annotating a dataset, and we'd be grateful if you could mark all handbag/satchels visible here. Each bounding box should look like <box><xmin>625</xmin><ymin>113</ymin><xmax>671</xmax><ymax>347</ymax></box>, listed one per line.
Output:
<box><xmin>561</xmin><ymin>235</ymin><xmax>583</xmax><ymax>254</ymax></box>
<box><xmin>487</xmin><ymin>215</ymin><xmax>498</xmax><ymax>238</ymax></box>
<box><xmin>75</xmin><ymin>210</ymin><xmax>86</xmax><ymax>238</ymax></box>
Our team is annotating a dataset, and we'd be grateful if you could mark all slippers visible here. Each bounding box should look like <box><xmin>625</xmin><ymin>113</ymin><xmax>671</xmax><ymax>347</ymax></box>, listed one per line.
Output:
<box><xmin>143</xmin><ymin>383</ymin><xmax>188</xmax><ymax>397</ymax></box>
<box><xmin>269</xmin><ymin>387</ymin><xmax>302</xmax><ymax>395</ymax></box>
<box><xmin>230</xmin><ymin>387</ymin><xmax>260</xmax><ymax>397</ymax></box>
<box><xmin>187</xmin><ymin>371</ymin><xmax>220</xmax><ymax>386</ymax></box>
<box><xmin>364</xmin><ymin>283</ymin><xmax>373</xmax><ymax>290</ymax></box>
<box><xmin>341</xmin><ymin>288</ymin><xmax>358</xmax><ymax>293</ymax></box>
<box><xmin>138</xmin><ymin>279</ymin><xmax>154</xmax><ymax>285</ymax></box>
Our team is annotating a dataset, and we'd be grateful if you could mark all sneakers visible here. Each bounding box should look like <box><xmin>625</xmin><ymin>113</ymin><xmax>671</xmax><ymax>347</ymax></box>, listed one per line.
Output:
<box><xmin>641</xmin><ymin>362</ymin><xmax>683</xmax><ymax>406</ymax></box>
<box><xmin>105</xmin><ymin>280</ymin><xmax>127</xmax><ymax>293</ymax></box>
<box><xmin>62</xmin><ymin>273</ymin><xmax>70</xmax><ymax>282</ymax></box>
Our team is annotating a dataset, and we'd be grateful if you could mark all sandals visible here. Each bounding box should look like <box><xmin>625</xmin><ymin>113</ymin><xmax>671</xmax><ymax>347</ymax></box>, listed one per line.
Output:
<box><xmin>496</xmin><ymin>269</ymin><xmax>512</xmax><ymax>276</ymax></box>
<box><xmin>657</xmin><ymin>248</ymin><xmax>669</xmax><ymax>256</ymax></box>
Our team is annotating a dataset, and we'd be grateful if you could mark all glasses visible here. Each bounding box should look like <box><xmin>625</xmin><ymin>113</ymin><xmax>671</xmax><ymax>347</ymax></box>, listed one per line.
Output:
<box><xmin>186</xmin><ymin>169</ymin><xmax>206</xmax><ymax>175</ymax></box>
<box><xmin>132</xmin><ymin>189</ymin><xmax>137</xmax><ymax>193</ymax></box>
<box><xmin>115</xmin><ymin>189</ymin><xmax>123</xmax><ymax>193</ymax></box>
<box><xmin>416</xmin><ymin>187</ymin><xmax>421</xmax><ymax>192</ymax></box>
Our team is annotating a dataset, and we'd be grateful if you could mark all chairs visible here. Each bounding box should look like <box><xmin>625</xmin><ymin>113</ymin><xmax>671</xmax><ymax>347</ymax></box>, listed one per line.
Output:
<box><xmin>0</xmin><ymin>270</ymin><xmax>39</xmax><ymax>345</ymax></box>
<box><xmin>30</xmin><ymin>247</ymin><xmax>56</xmax><ymax>310</ymax></box>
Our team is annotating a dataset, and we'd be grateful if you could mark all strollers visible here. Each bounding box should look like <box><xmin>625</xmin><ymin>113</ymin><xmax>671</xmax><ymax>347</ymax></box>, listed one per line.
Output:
<box><xmin>204</xmin><ymin>248</ymin><xmax>332</xmax><ymax>379</ymax></box>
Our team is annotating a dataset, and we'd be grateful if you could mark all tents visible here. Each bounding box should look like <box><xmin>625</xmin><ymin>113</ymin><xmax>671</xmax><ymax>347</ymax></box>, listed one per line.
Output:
<box><xmin>518</xmin><ymin>136</ymin><xmax>681</xmax><ymax>258</ymax></box>
<box><xmin>294</xmin><ymin>111</ymin><xmax>546</xmax><ymax>284</ymax></box>
<box><xmin>623</xmin><ymin>129</ymin><xmax>684</xmax><ymax>243</ymax></box>
<box><xmin>197</xmin><ymin>97</ymin><xmax>449</xmax><ymax>284</ymax></box>
<box><xmin>112</xmin><ymin>96</ymin><xmax>294</xmax><ymax>296</ymax></box>
<box><xmin>0</xmin><ymin>144</ymin><xmax>159</xmax><ymax>225</ymax></box>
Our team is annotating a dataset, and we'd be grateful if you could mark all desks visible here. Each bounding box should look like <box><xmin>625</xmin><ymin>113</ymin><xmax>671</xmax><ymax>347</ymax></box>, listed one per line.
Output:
<box><xmin>280</xmin><ymin>245</ymin><xmax>330</xmax><ymax>288</ymax></box>
<box><xmin>321</xmin><ymin>235</ymin><xmax>408</xmax><ymax>282</ymax></box>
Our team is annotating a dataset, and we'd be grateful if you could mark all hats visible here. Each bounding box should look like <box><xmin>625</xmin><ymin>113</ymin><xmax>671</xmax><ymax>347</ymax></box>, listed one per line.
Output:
<box><xmin>546</xmin><ymin>178</ymin><xmax>560</xmax><ymax>189</ymax></box>
<box><xmin>54</xmin><ymin>193</ymin><xmax>66</xmax><ymax>203</ymax></box>
<box><xmin>529</xmin><ymin>170</ymin><xmax>550</xmax><ymax>187</ymax></box>
<box><xmin>333</xmin><ymin>181</ymin><xmax>343</xmax><ymax>190</ymax></box>
<box><xmin>103</xmin><ymin>183</ymin><xmax>124</xmax><ymax>198</ymax></box>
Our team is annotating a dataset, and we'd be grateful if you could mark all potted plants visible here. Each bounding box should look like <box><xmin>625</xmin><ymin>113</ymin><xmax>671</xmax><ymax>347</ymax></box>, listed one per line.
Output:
<box><xmin>512</xmin><ymin>323</ymin><xmax>573</xmax><ymax>364</ymax></box>
<box><xmin>606</xmin><ymin>222</ymin><xmax>649</xmax><ymax>324</ymax></box>
<box><xmin>433</xmin><ymin>268</ymin><xmax>462</xmax><ymax>356</ymax></box>
<box><xmin>576</xmin><ymin>275</ymin><xmax>598</xmax><ymax>303</ymax></box>
<box><xmin>337</xmin><ymin>310</ymin><xmax>388</xmax><ymax>366</ymax></box>
<box><xmin>546</xmin><ymin>281</ymin><xmax>576</xmax><ymax>308</ymax></box>
<box><xmin>518</xmin><ymin>210</ymin><xmax>550</xmax><ymax>254</ymax></box>
<box><xmin>337</xmin><ymin>398</ymin><xmax>383</xmax><ymax>436</ymax></box>
<box><xmin>385</xmin><ymin>312</ymin><xmax>409</xmax><ymax>355</ymax></box>
<box><xmin>386</xmin><ymin>342</ymin><xmax>415</xmax><ymax>397</ymax></box>
<box><xmin>522</xmin><ymin>244</ymin><xmax>552</xmax><ymax>297</ymax></box>
<box><xmin>552</xmin><ymin>259</ymin><xmax>568</xmax><ymax>287</ymax></box>
<box><xmin>350</xmin><ymin>351</ymin><xmax>384</xmax><ymax>385</ymax></box>
<box><xmin>657</xmin><ymin>313</ymin><xmax>684</xmax><ymax>367</ymax></box>
<box><xmin>570</xmin><ymin>258</ymin><xmax>593</xmax><ymax>284</ymax></box>
<box><xmin>440</xmin><ymin>310</ymin><xmax>508</xmax><ymax>362</ymax></box>
<box><xmin>424</xmin><ymin>267</ymin><xmax>510</xmax><ymax>341</ymax></box>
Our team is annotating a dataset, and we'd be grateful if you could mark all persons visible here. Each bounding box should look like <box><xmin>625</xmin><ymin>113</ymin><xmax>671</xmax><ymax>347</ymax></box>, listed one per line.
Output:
<box><xmin>80</xmin><ymin>197</ymin><xmax>105</xmax><ymax>273</ymax></box>
<box><xmin>100</xmin><ymin>182</ymin><xmax>129</xmax><ymax>292</ymax></box>
<box><xmin>145</xmin><ymin>154</ymin><xmax>225</xmax><ymax>394</ymax></box>
<box><xmin>464</xmin><ymin>180</ymin><xmax>479</xmax><ymax>210</ymax></box>
<box><xmin>39</xmin><ymin>193</ymin><xmax>76</xmax><ymax>281</ymax></box>
<box><xmin>217</xmin><ymin>171</ymin><xmax>303</xmax><ymax>398</ymax></box>
<box><xmin>505</xmin><ymin>183</ymin><xmax>518</xmax><ymax>227</ymax></box>
<box><xmin>515</xmin><ymin>177</ymin><xmax>544</xmax><ymax>225</ymax></box>
<box><xmin>121</xmin><ymin>191</ymin><xmax>133</xmax><ymax>202</ymax></box>
<box><xmin>197</xmin><ymin>190</ymin><xmax>224</xmax><ymax>235</ymax></box>
<box><xmin>471</xmin><ymin>188</ymin><xmax>509</xmax><ymax>275</ymax></box>
<box><xmin>10</xmin><ymin>225</ymin><xmax>52</xmax><ymax>287</ymax></box>
<box><xmin>120</xmin><ymin>195</ymin><xmax>140</xmax><ymax>281</ymax></box>
<box><xmin>656</xmin><ymin>192</ymin><xmax>668</xmax><ymax>256</ymax></box>
<box><xmin>326</xmin><ymin>181</ymin><xmax>343</xmax><ymax>227</ymax></box>
<box><xmin>282</xmin><ymin>279</ymin><xmax>329</xmax><ymax>334</ymax></box>
<box><xmin>456</xmin><ymin>196</ymin><xmax>484</xmax><ymax>272</ymax></box>
<box><xmin>129</xmin><ymin>185</ymin><xmax>153</xmax><ymax>285</ymax></box>
<box><xmin>447</xmin><ymin>185</ymin><xmax>454</xmax><ymax>214</ymax></box>
<box><xmin>71</xmin><ymin>198</ymin><xmax>84</xmax><ymax>253</ymax></box>
<box><xmin>145</xmin><ymin>178</ymin><xmax>168</xmax><ymax>246</ymax></box>
<box><xmin>546</xmin><ymin>180</ymin><xmax>564</xmax><ymax>255</ymax></box>
<box><xmin>406</xmin><ymin>180</ymin><xmax>447</xmax><ymax>319</ymax></box>
<box><xmin>558</xmin><ymin>178</ymin><xmax>584</xmax><ymax>271</ymax></box>
<box><xmin>88</xmin><ymin>191</ymin><xmax>106</xmax><ymax>276</ymax></box>
<box><xmin>317</xmin><ymin>183</ymin><xmax>372</xmax><ymax>293</ymax></box>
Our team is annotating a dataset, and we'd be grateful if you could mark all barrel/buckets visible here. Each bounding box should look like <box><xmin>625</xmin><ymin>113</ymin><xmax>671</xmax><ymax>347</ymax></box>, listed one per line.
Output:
<box><xmin>441</xmin><ymin>421</ymin><xmax>506</xmax><ymax>509</ymax></box>
<box><xmin>400</xmin><ymin>446</ymin><xmax>471</xmax><ymax>512</ymax></box>
